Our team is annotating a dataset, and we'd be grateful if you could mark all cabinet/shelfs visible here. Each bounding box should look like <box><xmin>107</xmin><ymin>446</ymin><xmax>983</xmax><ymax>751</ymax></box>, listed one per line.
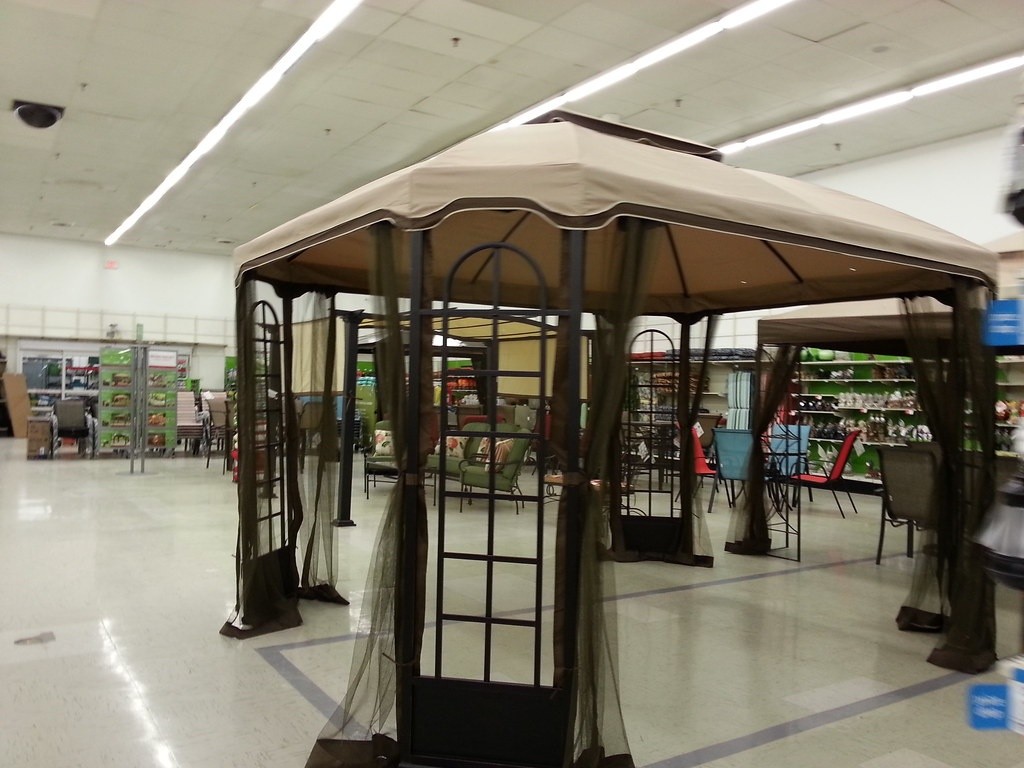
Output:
<box><xmin>792</xmin><ymin>358</ymin><xmax>1024</xmax><ymax>496</ymax></box>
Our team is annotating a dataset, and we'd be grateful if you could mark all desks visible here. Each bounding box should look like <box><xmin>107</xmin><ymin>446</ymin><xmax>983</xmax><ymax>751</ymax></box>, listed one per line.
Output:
<box><xmin>624</xmin><ymin>422</ymin><xmax>682</xmax><ymax>493</ymax></box>
<box><xmin>762</xmin><ymin>451</ymin><xmax>805</xmax><ymax>512</ymax></box>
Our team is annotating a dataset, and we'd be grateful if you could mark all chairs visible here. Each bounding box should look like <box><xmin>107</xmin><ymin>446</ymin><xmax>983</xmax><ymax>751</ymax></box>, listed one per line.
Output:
<box><xmin>875</xmin><ymin>440</ymin><xmax>1024</xmax><ymax>566</ymax></box>
<box><xmin>364</xmin><ymin>420</ymin><xmax>397</xmax><ymax>493</ymax></box>
<box><xmin>546</xmin><ymin>429</ymin><xmax>638</xmax><ymax>518</ymax></box>
<box><xmin>427</xmin><ymin>421</ymin><xmax>534</xmax><ymax>514</ymax></box>
<box><xmin>177</xmin><ymin>390</ymin><xmax>237</xmax><ymax>475</ymax></box>
<box><xmin>674</xmin><ymin>413</ymin><xmax>862</xmax><ymax>519</ymax></box>
<box><xmin>55</xmin><ymin>397</ymin><xmax>94</xmax><ymax>460</ymax></box>
<box><xmin>279</xmin><ymin>392</ymin><xmax>371</xmax><ymax>474</ymax></box>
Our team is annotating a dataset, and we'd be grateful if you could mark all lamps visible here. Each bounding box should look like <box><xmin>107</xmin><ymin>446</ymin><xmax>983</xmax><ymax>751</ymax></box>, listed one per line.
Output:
<box><xmin>480</xmin><ymin>0</ymin><xmax>1024</xmax><ymax>156</ymax></box>
<box><xmin>104</xmin><ymin>0</ymin><xmax>364</xmax><ymax>248</ymax></box>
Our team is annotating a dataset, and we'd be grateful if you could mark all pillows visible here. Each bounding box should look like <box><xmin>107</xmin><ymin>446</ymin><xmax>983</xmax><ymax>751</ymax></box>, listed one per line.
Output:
<box><xmin>485</xmin><ymin>438</ymin><xmax>514</xmax><ymax>472</ymax></box>
<box><xmin>434</xmin><ymin>430</ymin><xmax>470</xmax><ymax>459</ymax></box>
<box><xmin>476</xmin><ymin>436</ymin><xmax>500</xmax><ymax>462</ymax></box>
<box><xmin>374</xmin><ymin>430</ymin><xmax>395</xmax><ymax>456</ymax></box>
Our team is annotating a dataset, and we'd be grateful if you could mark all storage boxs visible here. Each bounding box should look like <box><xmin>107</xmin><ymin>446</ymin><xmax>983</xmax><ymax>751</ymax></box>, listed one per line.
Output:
<box><xmin>26</xmin><ymin>418</ymin><xmax>53</xmax><ymax>459</ymax></box>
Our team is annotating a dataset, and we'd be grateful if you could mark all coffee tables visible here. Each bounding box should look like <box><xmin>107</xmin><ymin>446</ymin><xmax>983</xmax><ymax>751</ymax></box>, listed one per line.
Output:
<box><xmin>366</xmin><ymin>459</ymin><xmax>438</xmax><ymax>506</ymax></box>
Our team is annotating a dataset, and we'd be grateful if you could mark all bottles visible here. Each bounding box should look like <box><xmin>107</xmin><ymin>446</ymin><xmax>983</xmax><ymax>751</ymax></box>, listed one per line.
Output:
<box><xmin>454</xmin><ymin>393</ymin><xmax>480</xmax><ymax>406</ymax></box>
<box><xmin>362</xmin><ymin>369</ymin><xmax>368</xmax><ymax>377</ymax></box>
<box><xmin>357</xmin><ymin>369</ymin><xmax>362</xmax><ymax>376</ymax></box>
<box><xmin>446</xmin><ymin>378</ymin><xmax>476</xmax><ymax>390</ymax></box>
<box><xmin>368</xmin><ymin>369</ymin><xmax>374</xmax><ymax>376</ymax></box>
<box><xmin>106</xmin><ymin>323</ymin><xmax>122</xmax><ymax>339</ymax></box>
